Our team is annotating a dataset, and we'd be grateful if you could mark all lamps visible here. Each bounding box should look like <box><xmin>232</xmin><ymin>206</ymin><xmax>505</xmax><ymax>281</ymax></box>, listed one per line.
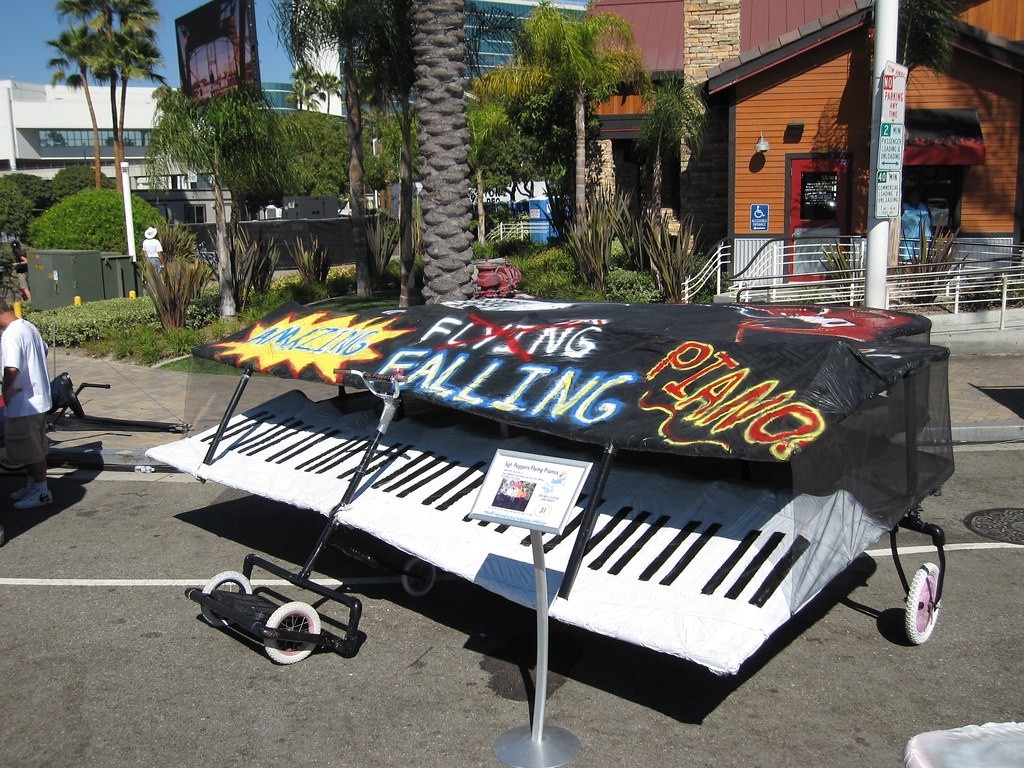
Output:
<box><xmin>752</xmin><ymin>130</ymin><xmax>769</xmax><ymax>153</ymax></box>
<box><xmin>788</xmin><ymin>122</ymin><xmax>804</xmax><ymax>134</ymax></box>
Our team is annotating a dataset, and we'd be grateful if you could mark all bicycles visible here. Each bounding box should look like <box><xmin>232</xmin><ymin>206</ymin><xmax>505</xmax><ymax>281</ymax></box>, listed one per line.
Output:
<box><xmin>0</xmin><ymin>257</ymin><xmax>28</xmax><ymax>308</ymax></box>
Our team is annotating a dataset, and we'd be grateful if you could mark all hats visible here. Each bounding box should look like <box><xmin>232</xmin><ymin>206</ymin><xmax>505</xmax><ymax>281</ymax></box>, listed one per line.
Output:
<box><xmin>144</xmin><ymin>226</ymin><xmax>157</xmax><ymax>239</ymax></box>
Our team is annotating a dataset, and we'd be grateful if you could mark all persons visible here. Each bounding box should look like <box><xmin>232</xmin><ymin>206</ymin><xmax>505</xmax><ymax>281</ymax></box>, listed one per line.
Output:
<box><xmin>10</xmin><ymin>241</ymin><xmax>31</xmax><ymax>300</ymax></box>
<box><xmin>901</xmin><ymin>189</ymin><xmax>932</xmax><ymax>260</ymax></box>
<box><xmin>142</xmin><ymin>227</ymin><xmax>164</xmax><ymax>280</ymax></box>
<box><xmin>0</xmin><ymin>299</ymin><xmax>54</xmax><ymax>509</ymax></box>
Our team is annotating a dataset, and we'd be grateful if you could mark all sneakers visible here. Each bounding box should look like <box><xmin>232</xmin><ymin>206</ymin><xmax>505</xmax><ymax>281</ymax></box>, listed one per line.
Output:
<box><xmin>11</xmin><ymin>487</ymin><xmax>53</xmax><ymax>508</ymax></box>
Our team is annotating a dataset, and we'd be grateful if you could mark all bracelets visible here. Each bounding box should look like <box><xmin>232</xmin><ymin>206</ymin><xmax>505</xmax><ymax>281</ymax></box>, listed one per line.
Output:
<box><xmin>19</xmin><ymin>262</ymin><xmax>21</xmax><ymax>265</ymax></box>
